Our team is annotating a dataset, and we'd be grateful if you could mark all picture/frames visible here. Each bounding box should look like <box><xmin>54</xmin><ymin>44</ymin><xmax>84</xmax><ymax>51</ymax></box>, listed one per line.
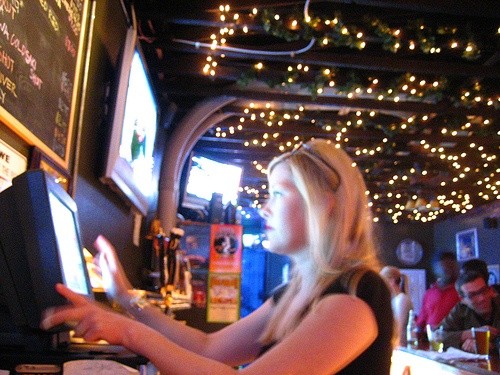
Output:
<box><xmin>456</xmin><ymin>226</ymin><xmax>479</xmax><ymax>262</ymax></box>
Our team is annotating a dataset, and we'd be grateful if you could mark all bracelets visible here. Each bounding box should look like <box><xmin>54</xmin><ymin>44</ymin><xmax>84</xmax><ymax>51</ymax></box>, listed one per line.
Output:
<box><xmin>126</xmin><ymin>296</ymin><xmax>150</xmax><ymax>314</ymax></box>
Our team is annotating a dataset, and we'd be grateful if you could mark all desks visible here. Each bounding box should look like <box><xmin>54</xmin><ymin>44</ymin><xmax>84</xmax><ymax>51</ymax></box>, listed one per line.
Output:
<box><xmin>389</xmin><ymin>346</ymin><xmax>500</xmax><ymax>375</ymax></box>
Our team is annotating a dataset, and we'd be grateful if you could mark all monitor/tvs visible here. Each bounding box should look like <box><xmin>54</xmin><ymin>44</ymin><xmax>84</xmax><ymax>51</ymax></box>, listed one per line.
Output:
<box><xmin>0</xmin><ymin>169</ymin><xmax>94</xmax><ymax>332</ymax></box>
<box><xmin>98</xmin><ymin>28</ymin><xmax>158</xmax><ymax>214</ymax></box>
<box><xmin>181</xmin><ymin>151</ymin><xmax>244</xmax><ymax>211</ymax></box>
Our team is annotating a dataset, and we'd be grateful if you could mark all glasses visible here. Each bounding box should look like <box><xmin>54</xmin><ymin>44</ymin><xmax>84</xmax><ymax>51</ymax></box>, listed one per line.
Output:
<box><xmin>291</xmin><ymin>140</ymin><xmax>342</xmax><ymax>190</ymax></box>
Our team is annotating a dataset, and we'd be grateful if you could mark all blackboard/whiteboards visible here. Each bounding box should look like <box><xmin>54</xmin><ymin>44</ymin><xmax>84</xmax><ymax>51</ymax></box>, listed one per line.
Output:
<box><xmin>0</xmin><ymin>0</ymin><xmax>92</xmax><ymax>176</ymax></box>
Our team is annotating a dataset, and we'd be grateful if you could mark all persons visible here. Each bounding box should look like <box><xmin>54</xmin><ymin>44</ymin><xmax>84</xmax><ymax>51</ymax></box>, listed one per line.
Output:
<box><xmin>414</xmin><ymin>252</ymin><xmax>500</xmax><ymax>354</ymax></box>
<box><xmin>41</xmin><ymin>139</ymin><xmax>395</xmax><ymax>375</ymax></box>
<box><xmin>379</xmin><ymin>266</ymin><xmax>415</xmax><ymax>346</ymax></box>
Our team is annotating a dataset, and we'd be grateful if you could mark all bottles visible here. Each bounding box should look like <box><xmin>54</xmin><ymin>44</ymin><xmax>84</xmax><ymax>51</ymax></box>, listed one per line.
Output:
<box><xmin>225</xmin><ymin>201</ymin><xmax>235</xmax><ymax>223</ymax></box>
<box><xmin>406</xmin><ymin>309</ymin><xmax>419</xmax><ymax>348</ymax></box>
<box><xmin>208</xmin><ymin>192</ymin><xmax>222</xmax><ymax>224</ymax></box>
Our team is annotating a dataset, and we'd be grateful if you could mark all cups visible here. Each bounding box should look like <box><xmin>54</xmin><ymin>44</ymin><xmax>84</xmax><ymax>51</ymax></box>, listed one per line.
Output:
<box><xmin>471</xmin><ymin>327</ymin><xmax>490</xmax><ymax>356</ymax></box>
<box><xmin>426</xmin><ymin>324</ymin><xmax>444</xmax><ymax>351</ymax></box>
